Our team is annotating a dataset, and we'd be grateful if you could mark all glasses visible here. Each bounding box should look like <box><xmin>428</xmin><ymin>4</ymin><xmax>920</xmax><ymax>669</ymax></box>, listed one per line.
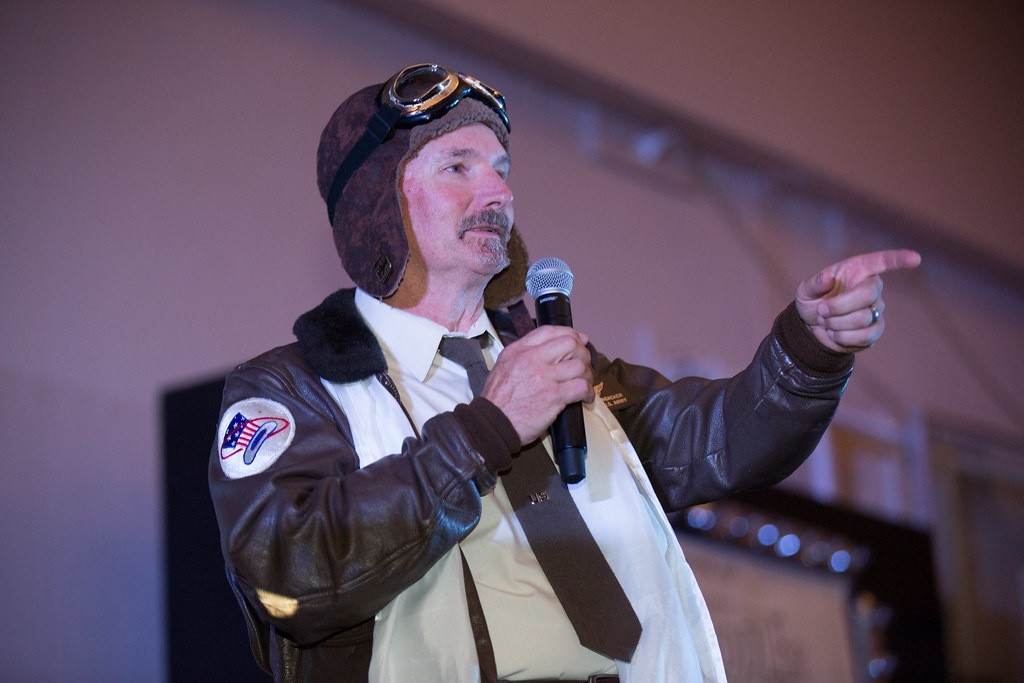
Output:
<box><xmin>376</xmin><ymin>61</ymin><xmax>511</xmax><ymax>129</ymax></box>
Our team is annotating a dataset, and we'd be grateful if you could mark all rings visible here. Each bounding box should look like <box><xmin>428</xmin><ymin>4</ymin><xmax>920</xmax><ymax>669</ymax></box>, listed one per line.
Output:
<box><xmin>870</xmin><ymin>305</ymin><xmax>879</xmax><ymax>322</ymax></box>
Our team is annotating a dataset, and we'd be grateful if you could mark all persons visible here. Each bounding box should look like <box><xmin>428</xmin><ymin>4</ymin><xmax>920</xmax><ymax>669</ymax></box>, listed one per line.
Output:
<box><xmin>210</xmin><ymin>65</ymin><xmax>921</xmax><ymax>683</ymax></box>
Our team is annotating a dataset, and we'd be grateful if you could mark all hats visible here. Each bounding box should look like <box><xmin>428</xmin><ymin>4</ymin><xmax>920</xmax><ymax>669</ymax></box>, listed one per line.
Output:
<box><xmin>317</xmin><ymin>83</ymin><xmax>531</xmax><ymax>310</ymax></box>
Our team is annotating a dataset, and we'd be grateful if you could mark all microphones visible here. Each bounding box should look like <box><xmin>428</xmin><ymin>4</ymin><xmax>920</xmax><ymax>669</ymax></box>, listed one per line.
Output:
<box><xmin>525</xmin><ymin>257</ymin><xmax>588</xmax><ymax>485</ymax></box>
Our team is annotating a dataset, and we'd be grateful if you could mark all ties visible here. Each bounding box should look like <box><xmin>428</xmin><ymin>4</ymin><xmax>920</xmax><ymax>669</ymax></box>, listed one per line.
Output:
<box><xmin>438</xmin><ymin>330</ymin><xmax>642</xmax><ymax>664</ymax></box>
